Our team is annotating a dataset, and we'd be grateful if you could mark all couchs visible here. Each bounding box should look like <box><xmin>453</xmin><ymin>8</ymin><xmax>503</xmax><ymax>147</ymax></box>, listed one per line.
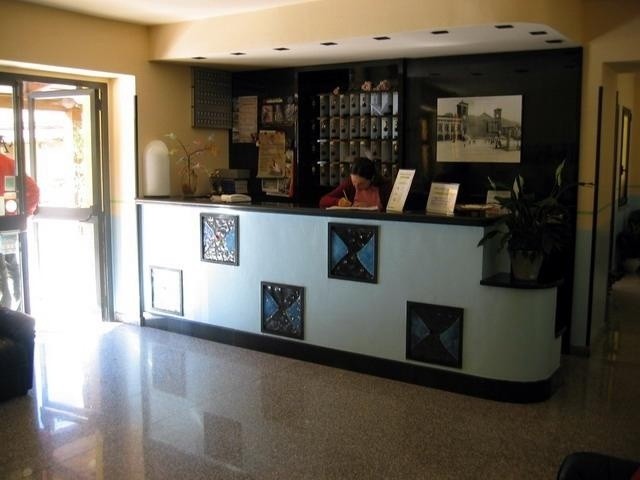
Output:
<box><xmin>0</xmin><ymin>307</ymin><xmax>35</xmax><ymax>401</ymax></box>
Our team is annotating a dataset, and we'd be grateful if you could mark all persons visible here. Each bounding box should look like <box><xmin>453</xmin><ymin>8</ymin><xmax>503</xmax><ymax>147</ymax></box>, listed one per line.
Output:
<box><xmin>319</xmin><ymin>157</ymin><xmax>393</xmax><ymax>212</ymax></box>
<box><xmin>0</xmin><ymin>152</ymin><xmax>38</xmax><ymax>310</ymax></box>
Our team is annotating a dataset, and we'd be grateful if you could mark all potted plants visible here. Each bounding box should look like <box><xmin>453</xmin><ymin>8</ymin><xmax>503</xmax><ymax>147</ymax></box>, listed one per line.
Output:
<box><xmin>476</xmin><ymin>159</ymin><xmax>594</xmax><ymax>282</ymax></box>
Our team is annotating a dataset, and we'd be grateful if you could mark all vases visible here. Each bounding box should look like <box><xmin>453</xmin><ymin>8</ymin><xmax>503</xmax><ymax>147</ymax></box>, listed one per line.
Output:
<box><xmin>181</xmin><ymin>170</ymin><xmax>197</xmax><ymax>201</ymax></box>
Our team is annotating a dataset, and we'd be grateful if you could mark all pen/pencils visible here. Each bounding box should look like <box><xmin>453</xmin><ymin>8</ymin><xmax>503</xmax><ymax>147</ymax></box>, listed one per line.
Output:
<box><xmin>343</xmin><ymin>189</ymin><xmax>348</xmax><ymax>201</ymax></box>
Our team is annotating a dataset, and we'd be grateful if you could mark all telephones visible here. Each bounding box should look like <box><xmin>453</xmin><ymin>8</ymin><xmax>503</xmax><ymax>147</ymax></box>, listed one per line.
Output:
<box><xmin>220</xmin><ymin>192</ymin><xmax>253</xmax><ymax>204</ymax></box>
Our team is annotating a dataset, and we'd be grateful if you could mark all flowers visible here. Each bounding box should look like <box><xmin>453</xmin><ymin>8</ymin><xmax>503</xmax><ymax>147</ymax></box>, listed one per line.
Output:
<box><xmin>165</xmin><ymin>132</ymin><xmax>217</xmax><ymax>170</ymax></box>
